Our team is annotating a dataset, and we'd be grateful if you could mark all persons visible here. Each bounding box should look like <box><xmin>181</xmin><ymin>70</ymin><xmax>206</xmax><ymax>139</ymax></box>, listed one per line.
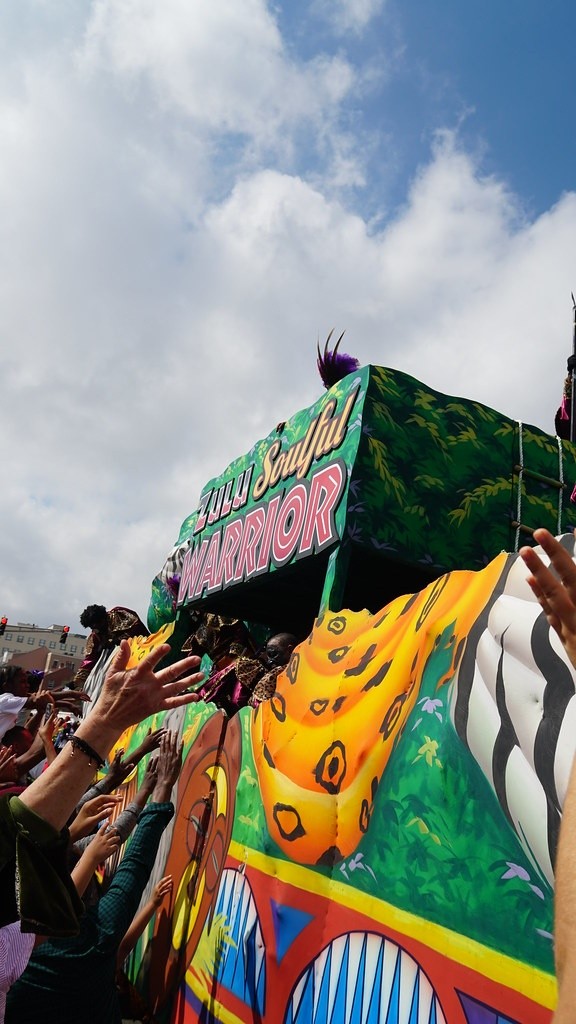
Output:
<box><xmin>0</xmin><ymin>605</ymin><xmax>298</xmax><ymax>1024</ymax></box>
<box><xmin>520</xmin><ymin>528</ymin><xmax>576</xmax><ymax>1024</ymax></box>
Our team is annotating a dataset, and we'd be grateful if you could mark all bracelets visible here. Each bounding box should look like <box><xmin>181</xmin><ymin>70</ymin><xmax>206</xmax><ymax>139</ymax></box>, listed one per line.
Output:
<box><xmin>124</xmin><ymin>809</ymin><xmax>138</xmax><ymax>817</ymax></box>
<box><xmin>91</xmin><ymin>784</ymin><xmax>104</xmax><ymax>794</ymax></box>
<box><xmin>66</xmin><ymin>735</ymin><xmax>105</xmax><ymax>771</ymax></box>
